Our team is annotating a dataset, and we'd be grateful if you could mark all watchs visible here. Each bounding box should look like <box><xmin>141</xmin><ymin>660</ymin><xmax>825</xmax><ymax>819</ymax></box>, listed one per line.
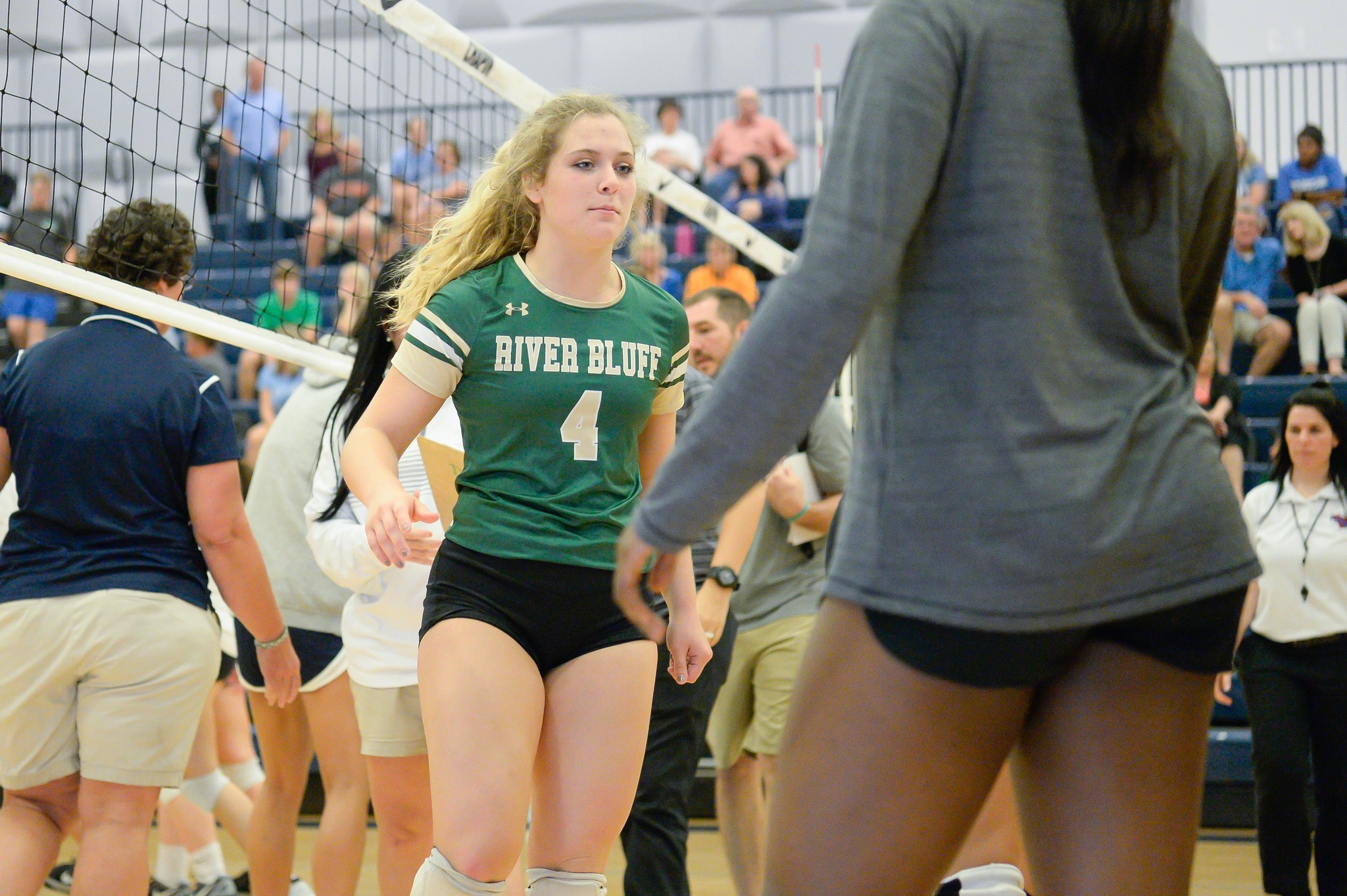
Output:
<box><xmin>702</xmin><ymin>566</ymin><xmax>740</xmax><ymax>590</ymax></box>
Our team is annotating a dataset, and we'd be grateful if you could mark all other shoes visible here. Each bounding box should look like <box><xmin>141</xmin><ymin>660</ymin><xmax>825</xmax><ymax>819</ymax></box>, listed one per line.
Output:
<box><xmin>43</xmin><ymin>864</ymin><xmax>74</xmax><ymax>896</ymax></box>
<box><xmin>288</xmin><ymin>879</ymin><xmax>317</xmax><ymax>896</ymax></box>
<box><xmin>233</xmin><ymin>871</ymin><xmax>251</xmax><ymax>893</ymax></box>
<box><xmin>150</xmin><ymin>879</ymin><xmax>193</xmax><ymax>896</ymax></box>
<box><xmin>192</xmin><ymin>876</ymin><xmax>238</xmax><ymax>896</ymax></box>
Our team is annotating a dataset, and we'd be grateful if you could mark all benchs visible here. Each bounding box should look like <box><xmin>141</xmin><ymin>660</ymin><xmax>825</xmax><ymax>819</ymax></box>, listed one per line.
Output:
<box><xmin>190</xmin><ymin>166</ymin><xmax>1347</xmax><ymax>835</ymax></box>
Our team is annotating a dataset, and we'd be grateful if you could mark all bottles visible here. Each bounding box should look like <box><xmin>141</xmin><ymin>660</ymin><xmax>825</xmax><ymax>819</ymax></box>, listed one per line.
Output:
<box><xmin>676</xmin><ymin>220</ymin><xmax>693</xmax><ymax>256</ymax></box>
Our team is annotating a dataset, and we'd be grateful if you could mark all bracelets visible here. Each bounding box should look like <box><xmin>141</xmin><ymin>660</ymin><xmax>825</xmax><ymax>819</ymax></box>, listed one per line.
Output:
<box><xmin>254</xmin><ymin>625</ymin><xmax>287</xmax><ymax>650</ymax></box>
<box><xmin>1329</xmin><ymin>285</ymin><xmax>1337</xmax><ymax>296</ymax></box>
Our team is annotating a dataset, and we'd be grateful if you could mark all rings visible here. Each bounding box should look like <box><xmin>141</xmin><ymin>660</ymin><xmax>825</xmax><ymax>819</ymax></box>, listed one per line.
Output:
<box><xmin>706</xmin><ymin>631</ymin><xmax>716</xmax><ymax>639</ymax></box>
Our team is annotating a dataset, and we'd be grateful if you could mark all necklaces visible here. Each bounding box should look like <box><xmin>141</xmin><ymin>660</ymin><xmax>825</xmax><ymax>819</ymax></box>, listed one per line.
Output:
<box><xmin>1307</xmin><ymin>257</ymin><xmax>1321</xmax><ymax>299</ymax></box>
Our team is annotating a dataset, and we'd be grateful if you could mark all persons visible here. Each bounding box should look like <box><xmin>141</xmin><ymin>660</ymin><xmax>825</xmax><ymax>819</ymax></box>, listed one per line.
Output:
<box><xmin>0</xmin><ymin>0</ymin><xmax>1347</xmax><ymax>896</ymax></box>
